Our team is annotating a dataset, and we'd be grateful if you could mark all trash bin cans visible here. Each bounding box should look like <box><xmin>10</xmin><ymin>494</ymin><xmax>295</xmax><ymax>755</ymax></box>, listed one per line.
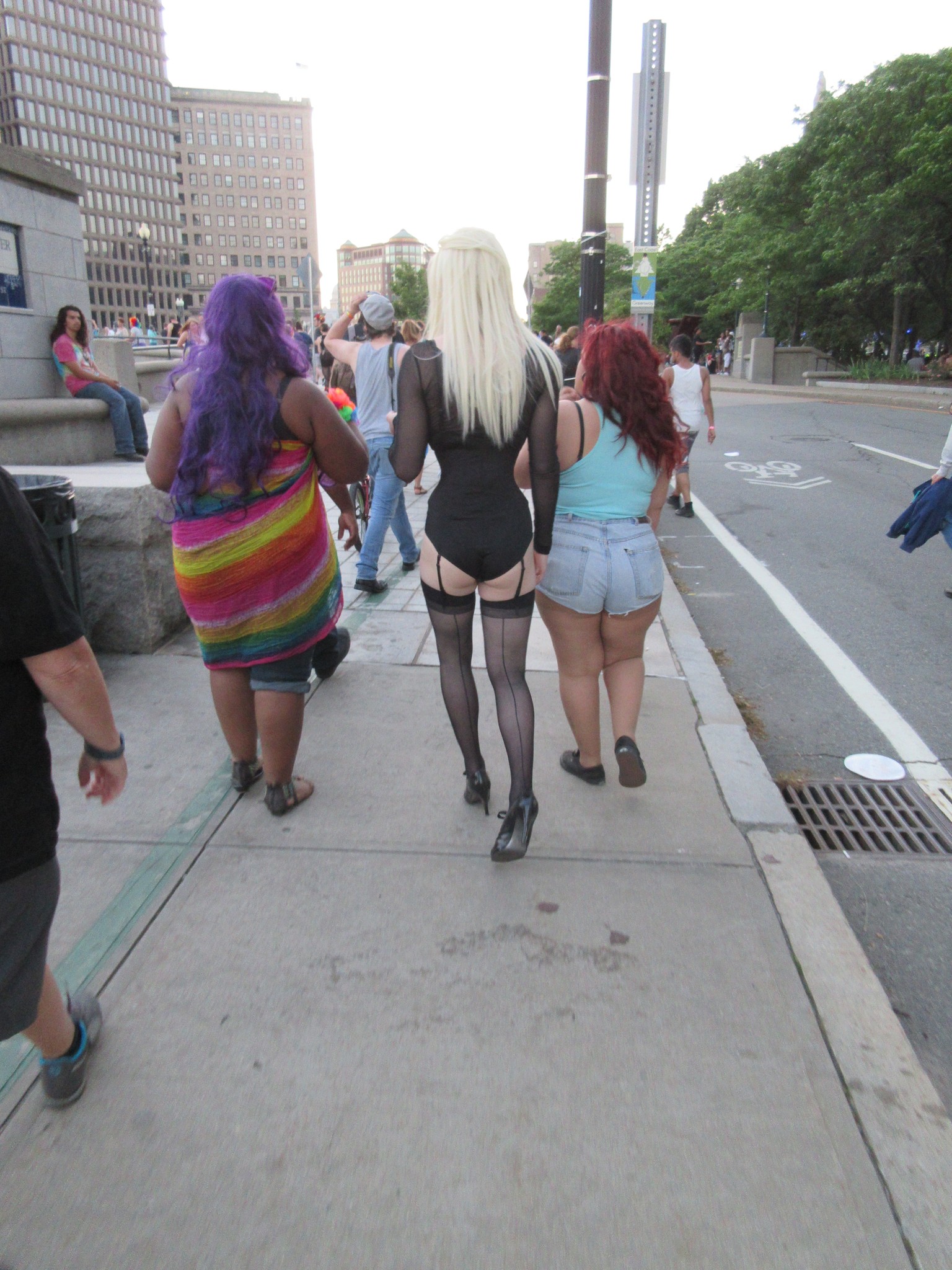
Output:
<box><xmin>8</xmin><ymin>474</ymin><xmax>83</xmax><ymax>688</ymax></box>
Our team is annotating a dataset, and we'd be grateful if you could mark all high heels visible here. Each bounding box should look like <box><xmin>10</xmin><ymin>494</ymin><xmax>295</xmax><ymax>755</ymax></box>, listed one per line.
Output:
<box><xmin>462</xmin><ymin>767</ymin><xmax>490</xmax><ymax>815</ymax></box>
<box><xmin>490</xmin><ymin>795</ymin><xmax>538</xmax><ymax>864</ymax></box>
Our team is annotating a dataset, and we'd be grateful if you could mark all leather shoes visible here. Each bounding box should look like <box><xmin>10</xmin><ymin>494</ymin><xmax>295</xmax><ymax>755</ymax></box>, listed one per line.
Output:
<box><xmin>353</xmin><ymin>578</ymin><xmax>388</xmax><ymax>593</ymax></box>
<box><xmin>401</xmin><ymin>547</ymin><xmax>422</xmax><ymax>571</ymax></box>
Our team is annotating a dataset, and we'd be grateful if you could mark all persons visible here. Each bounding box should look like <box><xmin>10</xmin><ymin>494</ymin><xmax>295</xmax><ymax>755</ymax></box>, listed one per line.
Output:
<box><xmin>50</xmin><ymin>304</ymin><xmax>150</xmax><ymax>464</ymax></box>
<box><xmin>511</xmin><ymin>311</ymin><xmax>692</xmax><ymax>792</ymax></box>
<box><xmin>309</xmin><ymin>467</ymin><xmax>361</xmax><ymax>681</ymax></box>
<box><xmin>660</xmin><ymin>331</ymin><xmax>716</xmax><ymax>518</ymax></box>
<box><xmin>930</xmin><ymin>412</ymin><xmax>952</xmax><ymax>602</ymax></box>
<box><xmin>0</xmin><ymin>465</ymin><xmax>134</xmax><ymax>1110</ymax></box>
<box><xmin>375</xmin><ymin>228</ymin><xmax>567</xmax><ymax>861</ymax></box>
<box><xmin>94</xmin><ymin>305</ymin><xmax>428</xmax><ymax>494</ymax></box>
<box><xmin>907</xmin><ymin>344</ymin><xmax>952</xmax><ymax>374</ymax></box>
<box><xmin>531</xmin><ymin>322</ymin><xmax>734</xmax><ymax>378</ymax></box>
<box><xmin>142</xmin><ymin>269</ymin><xmax>370</xmax><ymax>821</ymax></box>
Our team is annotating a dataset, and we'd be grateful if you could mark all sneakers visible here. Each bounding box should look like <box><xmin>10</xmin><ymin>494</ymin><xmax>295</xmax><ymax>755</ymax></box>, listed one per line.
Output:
<box><xmin>114</xmin><ymin>451</ymin><xmax>144</xmax><ymax>462</ymax></box>
<box><xmin>614</xmin><ymin>735</ymin><xmax>648</xmax><ymax>788</ymax></box>
<box><xmin>40</xmin><ymin>995</ymin><xmax>104</xmax><ymax>1108</ymax></box>
<box><xmin>136</xmin><ymin>448</ymin><xmax>149</xmax><ymax>456</ymax></box>
<box><xmin>716</xmin><ymin>369</ymin><xmax>730</xmax><ymax>376</ymax></box>
<box><xmin>561</xmin><ymin>749</ymin><xmax>605</xmax><ymax>786</ymax></box>
<box><xmin>943</xmin><ymin>587</ymin><xmax>952</xmax><ymax>598</ymax></box>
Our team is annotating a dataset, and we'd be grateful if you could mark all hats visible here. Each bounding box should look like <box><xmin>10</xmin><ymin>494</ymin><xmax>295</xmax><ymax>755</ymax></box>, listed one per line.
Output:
<box><xmin>359</xmin><ymin>291</ymin><xmax>395</xmax><ymax>330</ymax></box>
<box><xmin>729</xmin><ymin>331</ymin><xmax>734</xmax><ymax>336</ymax></box>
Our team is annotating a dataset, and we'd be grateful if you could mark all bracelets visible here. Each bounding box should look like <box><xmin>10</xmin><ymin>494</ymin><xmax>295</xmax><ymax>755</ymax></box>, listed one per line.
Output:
<box><xmin>82</xmin><ymin>730</ymin><xmax>125</xmax><ymax>761</ymax></box>
<box><xmin>708</xmin><ymin>426</ymin><xmax>715</xmax><ymax>431</ymax></box>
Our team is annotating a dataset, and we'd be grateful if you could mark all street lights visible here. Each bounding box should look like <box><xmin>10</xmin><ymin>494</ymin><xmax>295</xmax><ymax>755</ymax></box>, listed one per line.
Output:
<box><xmin>138</xmin><ymin>223</ymin><xmax>156</xmax><ymax>325</ymax></box>
<box><xmin>176</xmin><ymin>298</ymin><xmax>184</xmax><ymax>319</ymax></box>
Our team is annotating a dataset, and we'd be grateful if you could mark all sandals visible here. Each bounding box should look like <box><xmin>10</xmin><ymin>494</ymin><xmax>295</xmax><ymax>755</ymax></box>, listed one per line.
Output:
<box><xmin>265</xmin><ymin>776</ymin><xmax>314</xmax><ymax>815</ymax></box>
<box><xmin>414</xmin><ymin>486</ymin><xmax>427</xmax><ymax>495</ymax></box>
<box><xmin>230</xmin><ymin>756</ymin><xmax>263</xmax><ymax>792</ymax></box>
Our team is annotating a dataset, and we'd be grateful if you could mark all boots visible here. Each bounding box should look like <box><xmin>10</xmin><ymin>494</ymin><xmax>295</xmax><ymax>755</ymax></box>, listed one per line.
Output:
<box><xmin>675</xmin><ymin>502</ymin><xmax>694</xmax><ymax>517</ymax></box>
<box><xmin>666</xmin><ymin>496</ymin><xmax>680</xmax><ymax>509</ymax></box>
